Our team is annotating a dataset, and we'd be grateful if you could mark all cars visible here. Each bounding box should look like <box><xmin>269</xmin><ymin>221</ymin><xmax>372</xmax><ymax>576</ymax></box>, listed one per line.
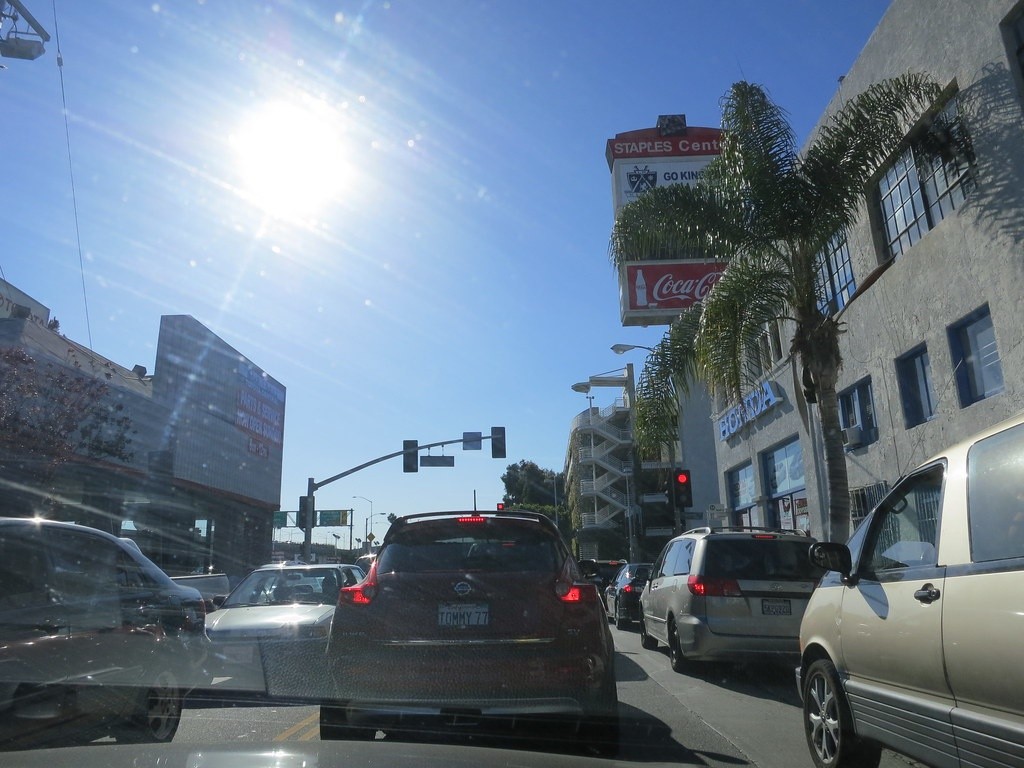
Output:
<box><xmin>167</xmin><ymin>549</ymin><xmax>375</xmax><ymax>677</ymax></box>
<box><xmin>600</xmin><ymin>561</ymin><xmax>656</xmax><ymax>630</ymax></box>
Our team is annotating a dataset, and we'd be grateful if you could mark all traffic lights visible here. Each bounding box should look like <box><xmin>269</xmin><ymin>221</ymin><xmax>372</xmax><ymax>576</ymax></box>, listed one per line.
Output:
<box><xmin>496</xmin><ymin>503</ymin><xmax>504</xmax><ymax>516</ymax></box>
<box><xmin>674</xmin><ymin>470</ymin><xmax>693</xmax><ymax>509</ymax></box>
<box><xmin>663</xmin><ymin>478</ymin><xmax>676</xmax><ymax>513</ymax></box>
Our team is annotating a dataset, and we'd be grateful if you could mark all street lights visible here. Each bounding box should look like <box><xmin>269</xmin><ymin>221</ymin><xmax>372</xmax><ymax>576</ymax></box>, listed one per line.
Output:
<box><xmin>622</xmin><ymin>466</ymin><xmax>636</xmax><ymax>562</ymax></box>
<box><xmin>352</xmin><ymin>495</ymin><xmax>373</xmax><ymax>554</ymax></box>
<box><xmin>609</xmin><ymin>338</ymin><xmax>684</xmax><ymax>537</ymax></box>
<box><xmin>543</xmin><ymin>475</ymin><xmax>559</xmax><ymax>527</ymax></box>
<box><xmin>365</xmin><ymin>512</ymin><xmax>387</xmax><ymax>553</ymax></box>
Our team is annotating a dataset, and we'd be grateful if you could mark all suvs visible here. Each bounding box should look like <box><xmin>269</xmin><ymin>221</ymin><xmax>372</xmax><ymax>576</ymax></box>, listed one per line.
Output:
<box><xmin>578</xmin><ymin>557</ymin><xmax>628</xmax><ymax>599</ymax></box>
<box><xmin>0</xmin><ymin>515</ymin><xmax>213</xmax><ymax>752</ymax></box>
<box><xmin>634</xmin><ymin>527</ymin><xmax>831</xmax><ymax>680</ymax></box>
<box><xmin>794</xmin><ymin>412</ymin><xmax>1023</xmax><ymax>768</ymax></box>
<box><xmin>318</xmin><ymin>511</ymin><xmax>622</xmax><ymax>757</ymax></box>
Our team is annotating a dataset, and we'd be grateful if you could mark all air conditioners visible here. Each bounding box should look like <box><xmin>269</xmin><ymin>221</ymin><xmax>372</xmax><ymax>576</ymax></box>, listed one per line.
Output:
<box><xmin>841</xmin><ymin>426</ymin><xmax>862</xmax><ymax>448</ymax></box>
